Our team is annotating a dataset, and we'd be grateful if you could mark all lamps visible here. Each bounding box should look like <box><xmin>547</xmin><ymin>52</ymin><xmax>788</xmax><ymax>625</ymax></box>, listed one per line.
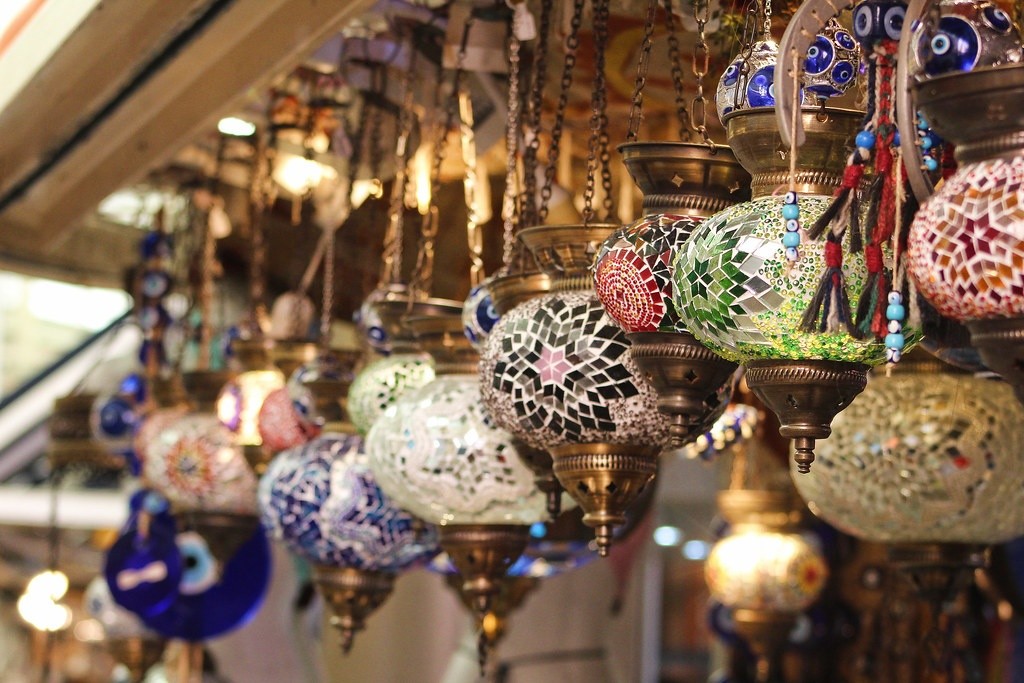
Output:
<box><xmin>1</xmin><ymin>1</ymin><xmax>1024</xmax><ymax>683</ymax></box>
